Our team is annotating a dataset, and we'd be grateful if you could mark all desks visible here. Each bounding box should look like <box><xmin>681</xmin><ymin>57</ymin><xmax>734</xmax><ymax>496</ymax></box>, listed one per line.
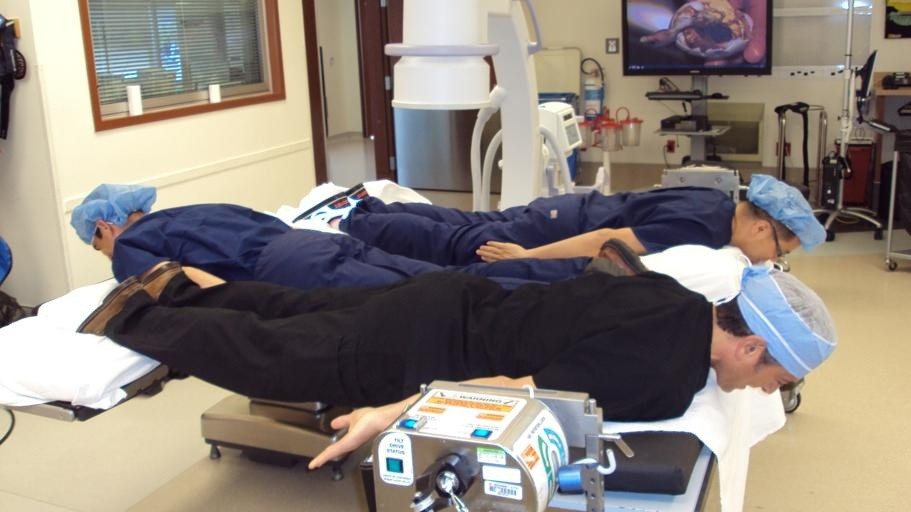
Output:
<box><xmin>871</xmin><ymin>72</ymin><xmax>911</xmax><ymax>213</ymax></box>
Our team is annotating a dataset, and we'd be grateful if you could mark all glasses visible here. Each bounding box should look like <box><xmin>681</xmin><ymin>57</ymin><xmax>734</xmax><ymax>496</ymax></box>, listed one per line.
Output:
<box><xmin>766</xmin><ymin>219</ymin><xmax>791</xmax><ymax>272</ymax></box>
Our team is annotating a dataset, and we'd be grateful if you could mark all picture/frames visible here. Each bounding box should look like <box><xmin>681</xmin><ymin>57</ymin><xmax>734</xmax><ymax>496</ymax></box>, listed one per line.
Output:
<box><xmin>884</xmin><ymin>0</ymin><xmax>911</xmax><ymax>39</ymax></box>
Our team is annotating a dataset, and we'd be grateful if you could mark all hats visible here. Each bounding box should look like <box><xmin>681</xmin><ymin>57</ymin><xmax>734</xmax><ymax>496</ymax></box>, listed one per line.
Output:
<box><xmin>70</xmin><ymin>183</ymin><xmax>157</xmax><ymax>246</ymax></box>
<box><xmin>737</xmin><ymin>264</ymin><xmax>836</xmax><ymax>379</ymax></box>
<box><xmin>746</xmin><ymin>172</ymin><xmax>826</xmax><ymax>253</ymax></box>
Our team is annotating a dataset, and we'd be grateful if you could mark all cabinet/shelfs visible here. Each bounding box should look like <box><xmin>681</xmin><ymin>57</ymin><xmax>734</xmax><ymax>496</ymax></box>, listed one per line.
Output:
<box><xmin>648</xmin><ymin>94</ymin><xmax>731</xmax><ymax>167</ymax></box>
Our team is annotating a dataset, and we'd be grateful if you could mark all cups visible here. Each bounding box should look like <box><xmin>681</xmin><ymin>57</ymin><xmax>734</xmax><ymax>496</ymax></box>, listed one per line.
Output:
<box><xmin>207</xmin><ymin>84</ymin><xmax>221</xmax><ymax>103</ymax></box>
<box><xmin>125</xmin><ymin>84</ymin><xmax>142</xmax><ymax>116</ymax></box>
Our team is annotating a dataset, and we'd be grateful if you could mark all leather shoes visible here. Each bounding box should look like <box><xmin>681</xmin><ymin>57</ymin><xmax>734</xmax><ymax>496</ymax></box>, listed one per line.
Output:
<box><xmin>585</xmin><ymin>238</ymin><xmax>646</xmax><ymax>275</ymax></box>
<box><xmin>76</xmin><ymin>275</ymin><xmax>145</xmax><ymax>336</ymax></box>
<box><xmin>139</xmin><ymin>261</ymin><xmax>183</xmax><ymax>301</ymax></box>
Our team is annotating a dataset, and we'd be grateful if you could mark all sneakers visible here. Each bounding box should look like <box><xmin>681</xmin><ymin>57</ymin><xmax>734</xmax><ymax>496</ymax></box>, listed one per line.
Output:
<box><xmin>345</xmin><ymin>183</ymin><xmax>369</xmax><ymax>208</ymax></box>
<box><xmin>292</xmin><ymin>191</ymin><xmax>352</xmax><ymax>224</ymax></box>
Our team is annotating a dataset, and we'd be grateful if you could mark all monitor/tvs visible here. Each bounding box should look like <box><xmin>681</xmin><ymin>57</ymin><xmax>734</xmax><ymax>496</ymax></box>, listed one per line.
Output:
<box><xmin>621</xmin><ymin>0</ymin><xmax>774</xmax><ymax>77</ymax></box>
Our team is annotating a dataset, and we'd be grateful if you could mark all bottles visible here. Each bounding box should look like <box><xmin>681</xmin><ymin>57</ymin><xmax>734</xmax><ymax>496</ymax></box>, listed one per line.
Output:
<box><xmin>578</xmin><ymin>117</ymin><xmax>643</xmax><ymax>152</ymax></box>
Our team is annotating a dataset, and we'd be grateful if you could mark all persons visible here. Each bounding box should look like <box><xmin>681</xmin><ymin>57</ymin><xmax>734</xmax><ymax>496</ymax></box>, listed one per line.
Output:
<box><xmin>76</xmin><ymin>261</ymin><xmax>839</xmax><ymax>469</ymax></box>
<box><xmin>291</xmin><ymin>173</ymin><xmax>822</xmax><ymax>263</ymax></box>
<box><xmin>69</xmin><ymin>183</ymin><xmax>646</xmax><ymax>291</ymax></box>
